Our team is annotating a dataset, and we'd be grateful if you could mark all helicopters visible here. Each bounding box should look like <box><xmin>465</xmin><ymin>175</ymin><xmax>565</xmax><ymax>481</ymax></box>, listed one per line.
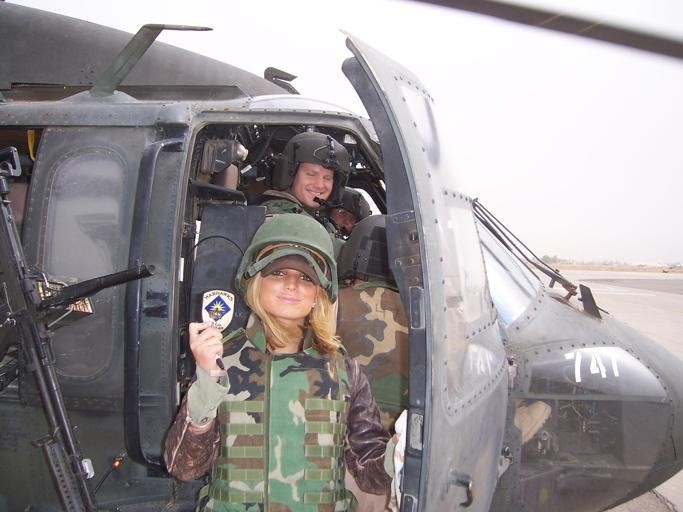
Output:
<box><xmin>0</xmin><ymin>0</ymin><xmax>683</xmax><ymax>511</ymax></box>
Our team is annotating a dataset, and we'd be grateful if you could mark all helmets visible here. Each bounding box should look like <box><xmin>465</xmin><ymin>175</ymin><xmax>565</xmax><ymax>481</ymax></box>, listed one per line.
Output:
<box><xmin>272</xmin><ymin>131</ymin><xmax>351</xmax><ymax>205</ymax></box>
<box><xmin>234</xmin><ymin>212</ymin><xmax>339</xmax><ymax>305</ymax></box>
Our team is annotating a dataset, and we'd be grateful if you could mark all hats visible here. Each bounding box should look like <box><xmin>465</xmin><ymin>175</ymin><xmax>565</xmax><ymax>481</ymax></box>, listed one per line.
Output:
<box><xmin>260</xmin><ymin>246</ymin><xmax>325</xmax><ymax>286</ymax></box>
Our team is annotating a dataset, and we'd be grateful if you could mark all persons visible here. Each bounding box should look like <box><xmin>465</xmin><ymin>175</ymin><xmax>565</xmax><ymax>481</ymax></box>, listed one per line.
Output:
<box><xmin>260</xmin><ymin>132</ymin><xmax>553</xmax><ymax>445</ymax></box>
<box><xmin>164</xmin><ymin>212</ymin><xmax>400</xmax><ymax>512</ymax></box>
<box><xmin>334</xmin><ymin>214</ymin><xmax>409</xmax><ymax>436</ymax></box>
<box><xmin>329</xmin><ymin>187</ymin><xmax>373</xmax><ymax>234</ymax></box>
